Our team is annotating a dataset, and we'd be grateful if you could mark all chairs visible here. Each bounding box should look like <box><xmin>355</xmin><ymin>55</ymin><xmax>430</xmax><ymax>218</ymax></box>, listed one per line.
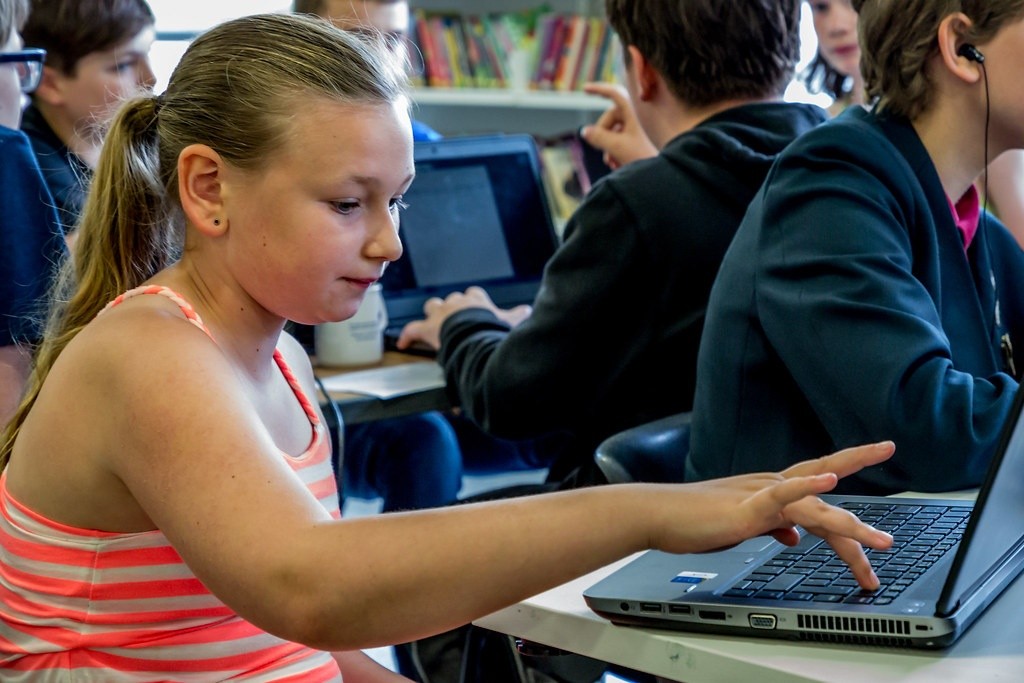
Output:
<box><xmin>368</xmin><ymin>411</ymin><xmax>522</xmax><ymax>683</ymax></box>
<box><xmin>593</xmin><ymin>412</ymin><xmax>692</xmax><ymax>484</ymax></box>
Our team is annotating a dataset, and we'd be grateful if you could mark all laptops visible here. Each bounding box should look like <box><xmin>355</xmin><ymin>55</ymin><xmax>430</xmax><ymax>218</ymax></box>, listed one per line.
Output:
<box><xmin>581</xmin><ymin>380</ymin><xmax>1024</xmax><ymax>650</ymax></box>
<box><xmin>375</xmin><ymin>132</ymin><xmax>563</xmax><ymax>355</ymax></box>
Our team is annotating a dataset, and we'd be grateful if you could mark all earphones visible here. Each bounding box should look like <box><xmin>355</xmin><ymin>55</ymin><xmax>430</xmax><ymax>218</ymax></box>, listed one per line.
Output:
<box><xmin>957</xmin><ymin>43</ymin><xmax>986</xmax><ymax>65</ymax></box>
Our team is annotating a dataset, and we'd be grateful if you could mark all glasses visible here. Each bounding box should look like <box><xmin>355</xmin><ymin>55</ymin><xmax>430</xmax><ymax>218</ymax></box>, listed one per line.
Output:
<box><xmin>0</xmin><ymin>48</ymin><xmax>47</xmax><ymax>94</ymax></box>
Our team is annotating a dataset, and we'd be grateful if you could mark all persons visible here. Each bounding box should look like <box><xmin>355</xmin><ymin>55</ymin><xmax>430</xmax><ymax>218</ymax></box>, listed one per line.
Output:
<box><xmin>400</xmin><ymin>0</ymin><xmax>828</xmax><ymax>491</ymax></box>
<box><xmin>0</xmin><ymin>0</ymin><xmax>69</xmax><ymax>442</ymax></box>
<box><xmin>17</xmin><ymin>0</ymin><xmax>188</xmax><ymax>283</ymax></box>
<box><xmin>293</xmin><ymin>0</ymin><xmax>554</xmax><ymax>515</ymax></box>
<box><xmin>684</xmin><ymin>0</ymin><xmax>1024</xmax><ymax>496</ymax></box>
<box><xmin>808</xmin><ymin>0</ymin><xmax>1024</xmax><ymax>250</ymax></box>
<box><xmin>0</xmin><ymin>12</ymin><xmax>896</xmax><ymax>682</ymax></box>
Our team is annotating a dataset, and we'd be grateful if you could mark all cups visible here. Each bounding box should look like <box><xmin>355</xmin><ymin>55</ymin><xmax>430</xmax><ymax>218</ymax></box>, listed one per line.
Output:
<box><xmin>314</xmin><ymin>283</ymin><xmax>387</xmax><ymax>366</ymax></box>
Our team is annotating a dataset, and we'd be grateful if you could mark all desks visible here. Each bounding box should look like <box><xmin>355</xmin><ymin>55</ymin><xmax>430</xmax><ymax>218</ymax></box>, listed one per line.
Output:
<box><xmin>310</xmin><ymin>349</ymin><xmax>452</xmax><ymax>429</ymax></box>
<box><xmin>471</xmin><ymin>487</ymin><xmax>1024</xmax><ymax>683</ymax></box>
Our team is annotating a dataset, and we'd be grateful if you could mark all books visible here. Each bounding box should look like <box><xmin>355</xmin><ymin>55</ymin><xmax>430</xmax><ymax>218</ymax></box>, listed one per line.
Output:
<box><xmin>407</xmin><ymin>10</ymin><xmax>627</xmax><ymax>219</ymax></box>
